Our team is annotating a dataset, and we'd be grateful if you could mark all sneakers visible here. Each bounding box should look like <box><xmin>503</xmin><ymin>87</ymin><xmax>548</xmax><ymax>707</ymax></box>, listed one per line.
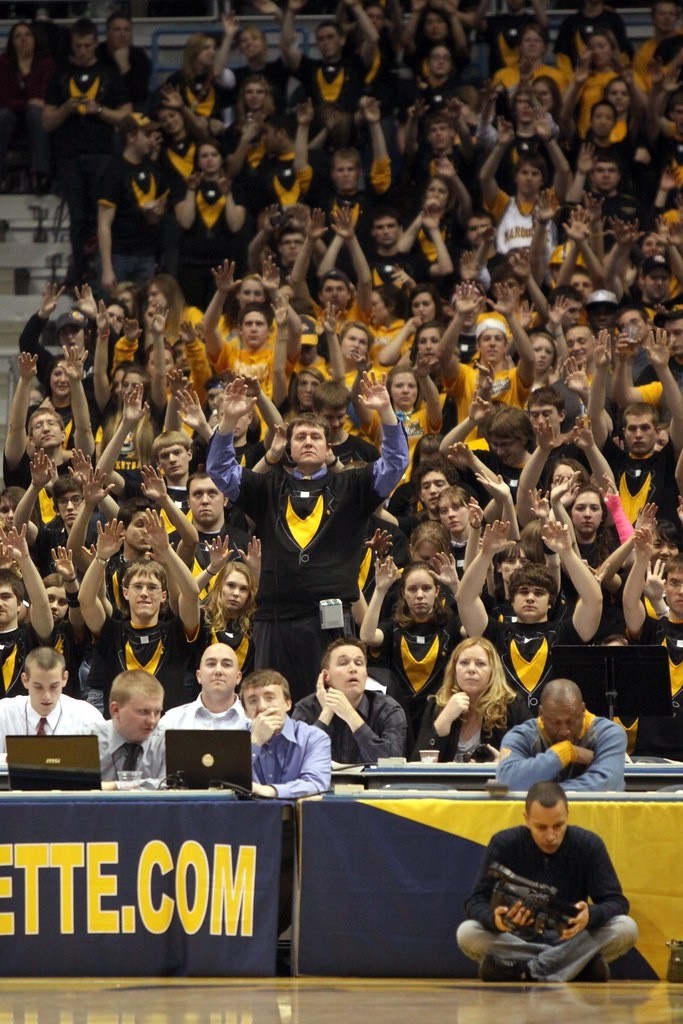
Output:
<box><xmin>574</xmin><ymin>954</ymin><xmax>610</xmax><ymax>982</ymax></box>
<box><xmin>479</xmin><ymin>954</ymin><xmax>534</xmax><ymax>981</ymax></box>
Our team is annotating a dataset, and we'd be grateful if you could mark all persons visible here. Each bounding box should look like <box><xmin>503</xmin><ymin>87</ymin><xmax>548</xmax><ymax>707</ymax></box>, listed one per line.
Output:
<box><xmin>454</xmin><ymin>780</ymin><xmax>639</xmax><ymax>979</ymax></box>
<box><xmin>0</xmin><ymin>0</ymin><xmax>683</xmax><ymax>791</ymax></box>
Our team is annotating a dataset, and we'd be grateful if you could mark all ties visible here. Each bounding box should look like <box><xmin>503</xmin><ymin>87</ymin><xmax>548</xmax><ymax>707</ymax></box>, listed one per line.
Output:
<box><xmin>37</xmin><ymin>718</ymin><xmax>48</xmax><ymax>736</ymax></box>
<box><xmin>122</xmin><ymin>743</ymin><xmax>141</xmax><ymax>770</ymax></box>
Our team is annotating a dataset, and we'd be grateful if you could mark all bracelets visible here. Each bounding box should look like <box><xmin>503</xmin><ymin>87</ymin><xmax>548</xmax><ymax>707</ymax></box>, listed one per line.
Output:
<box><xmin>470</xmin><ymin>522</ymin><xmax>482</xmax><ymax>529</ymax></box>
<box><xmin>326</xmin><ymin>457</ymin><xmax>339</xmax><ymax>468</ymax></box>
<box><xmin>656</xmin><ymin>607</ymin><xmax>669</xmax><ymax>615</ymax></box>
<box><xmin>264</xmin><ymin>450</ymin><xmax>283</xmax><ymax>466</ymax></box>
<box><xmin>63</xmin><ymin>576</ymin><xmax>77</xmax><ymax>582</ymax></box>
<box><xmin>65</xmin><ymin>588</ymin><xmax>80</xmax><ymax>608</ymax></box>
<box><xmin>543</xmin><ymin>135</ymin><xmax>554</xmax><ymax>142</ymax></box>
<box><xmin>96</xmin><ymin>552</ymin><xmax>110</xmax><ymax>564</ymax></box>
<box><xmin>98</xmin><ymin>328</ymin><xmax>113</xmax><ymax>339</ymax></box>
<box><xmin>206</xmin><ymin>567</ymin><xmax>216</xmax><ymax>575</ymax></box>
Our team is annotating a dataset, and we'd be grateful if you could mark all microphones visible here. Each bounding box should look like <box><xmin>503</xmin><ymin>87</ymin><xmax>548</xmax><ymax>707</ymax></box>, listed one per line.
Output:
<box><xmin>529</xmin><ymin>893</ymin><xmax>580</xmax><ymax>919</ymax></box>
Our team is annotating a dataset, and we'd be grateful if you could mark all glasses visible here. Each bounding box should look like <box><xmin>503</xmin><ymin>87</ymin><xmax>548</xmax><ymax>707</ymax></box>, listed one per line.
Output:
<box><xmin>490</xmin><ymin>439</ymin><xmax>518</xmax><ymax>447</ymax></box>
<box><xmin>56</xmin><ymin>495</ymin><xmax>84</xmax><ymax>506</ymax></box>
<box><xmin>128</xmin><ymin>583</ymin><xmax>163</xmax><ymax>595</ymax></box>
<box><xmin>668</xmin><ymin>581</ymin><xmax>683</xmax><ymax>587</ymax></box>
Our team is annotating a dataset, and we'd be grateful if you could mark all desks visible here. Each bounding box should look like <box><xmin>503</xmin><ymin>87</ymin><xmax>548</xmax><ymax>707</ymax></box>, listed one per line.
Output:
<box><xmin>1</xmin><ymin>798</ymin><xmax>290</xmax><ymax>979</ymax></box>
<box><xmin>329</xmin><ymin>765</ymin><xmax>683</xmax><ymax>791</ymax></box>
<box><xmin>293</xmin><ymin>792</ymin><xmax>683</xmax><ymax>982</ymax></box>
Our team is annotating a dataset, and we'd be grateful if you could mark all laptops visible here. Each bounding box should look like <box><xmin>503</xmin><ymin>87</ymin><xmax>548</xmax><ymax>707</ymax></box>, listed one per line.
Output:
<box><xmin>5</xmin><ymin>735</ymin><xmax>102</xmax><ymax>793</ymax></box>
<box><xmin>165</xmin><ymin>730</ymin><xmax>255</xmax><ymax>802</ymax></box>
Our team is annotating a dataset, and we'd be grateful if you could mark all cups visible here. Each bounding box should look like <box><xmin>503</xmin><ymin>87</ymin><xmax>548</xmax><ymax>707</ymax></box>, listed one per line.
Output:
<box><xmin>624</xmin><ymin>324</ymin><xmax>640</xmax><ymax>353</ymax></box>
<box><xmin>117</xmin><ymin>771</ymin><xmax>143</xmax><ymax>789</ymax></box>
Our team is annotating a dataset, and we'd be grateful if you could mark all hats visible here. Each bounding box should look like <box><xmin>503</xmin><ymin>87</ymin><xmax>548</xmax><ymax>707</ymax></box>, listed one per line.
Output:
<box><xmin>477</xmin><ymin>319</ymin><xmax>506</xmax><ymax>339</ymax></box>
<box><xmin>653</xmin><ymin>309</ymin><xmax>683</xmax><ymax>328</ymax></box>
<box><xmin>120</xmin><ymin>112</ymin><xmax>161</xmax><ymax>137</ymax></box>
<box><xmin>55</xmin><ymin>312</ymin><xmax>88</xmax><ymax>337</ymax></box>
<box><xmin>319</xmin><ymin>269</ymin><xmax>349</xmax><ymax>288</ymax></box>
<box><xmin>642</xmin><ymin>255</ymin><xmax>671</xmax><ymax>276</ymax></box>
<box><xmin>585</xmin><ymin>289</ymin><xmax>619</xmax><ymax>308</ymax></box>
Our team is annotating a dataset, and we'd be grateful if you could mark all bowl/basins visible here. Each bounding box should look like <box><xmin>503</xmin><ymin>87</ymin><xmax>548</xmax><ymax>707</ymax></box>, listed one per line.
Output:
<box><xmin>419</xmin><ymin>750</ymin><xmax>439</xmax><ymax>762</ymax></box>
